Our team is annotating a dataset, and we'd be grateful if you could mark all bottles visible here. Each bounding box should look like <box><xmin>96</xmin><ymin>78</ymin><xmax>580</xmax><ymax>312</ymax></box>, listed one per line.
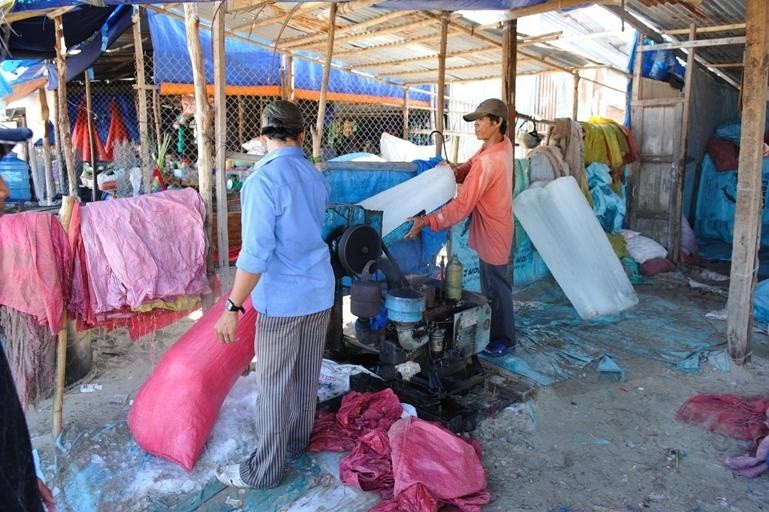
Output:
<box><xmin>445</xmin><ymin>254</ymin><xmax>462</xmax><ymax>301</ymax></box>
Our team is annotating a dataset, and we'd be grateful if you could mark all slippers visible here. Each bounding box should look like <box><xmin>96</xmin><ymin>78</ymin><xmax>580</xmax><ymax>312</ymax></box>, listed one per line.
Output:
<box><xmin>481</xmin><ymin>340</ymin><xmax>516</xmax><ymax>357</ymax></box>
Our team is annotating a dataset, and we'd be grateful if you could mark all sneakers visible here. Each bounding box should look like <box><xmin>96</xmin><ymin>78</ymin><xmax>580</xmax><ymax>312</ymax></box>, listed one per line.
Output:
<box><xmin>216</xmin><ymin>463</ymin><xmax>252</xmax><ymax>489</ymax></box>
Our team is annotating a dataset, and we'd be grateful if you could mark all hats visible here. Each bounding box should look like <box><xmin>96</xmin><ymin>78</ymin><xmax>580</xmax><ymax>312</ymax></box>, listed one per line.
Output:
<box><xmin>464</xmin><ymin>98</ymin><xmax>508</xmax><ymax>121</ymax></box>
<box><xmin>261</xmin><ymin>100</ymin><xmax>306</xmax><ymax>128</ymax></box>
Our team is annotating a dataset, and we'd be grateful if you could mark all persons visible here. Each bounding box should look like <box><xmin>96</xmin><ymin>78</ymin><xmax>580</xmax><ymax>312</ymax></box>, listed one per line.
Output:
<box><xmin>402</xmin><ymin>99</ymin><xmax>518</xmax><ymax>356</ymax></box>
<box><xmin>0</xmin><ymin>343</ymin><xmax>58</xmax><ymax>512</ymax></box>
<box><xmin>213</xmin><ymin>99</ymin><xmax>337</xmax><ymax>490</ymax></box>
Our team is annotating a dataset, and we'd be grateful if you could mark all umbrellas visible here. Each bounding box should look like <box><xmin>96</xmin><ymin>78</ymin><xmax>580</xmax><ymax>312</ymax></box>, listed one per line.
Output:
<box><xmin>104</xmin><ymin>95</ymin><xmax>131</xmax><ymax>162</ymax></box>
<box><xmin>68</xmin><ymin>86</ymin><xmax>111</xmax><ymax>164</ymax></box>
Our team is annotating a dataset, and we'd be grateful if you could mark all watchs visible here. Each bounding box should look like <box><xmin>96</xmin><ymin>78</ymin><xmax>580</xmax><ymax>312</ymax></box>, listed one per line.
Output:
<box><xmin>223</xmin><ymin>297</ymin><xmax>246</xmax><ymax>314</ymax></box>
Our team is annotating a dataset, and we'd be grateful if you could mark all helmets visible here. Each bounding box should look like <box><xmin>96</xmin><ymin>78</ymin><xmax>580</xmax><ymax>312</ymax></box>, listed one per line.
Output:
<box><xmin>518</xmin><ymin>131</ymin><xmax>545</xmax><ymax>148</ymax></box>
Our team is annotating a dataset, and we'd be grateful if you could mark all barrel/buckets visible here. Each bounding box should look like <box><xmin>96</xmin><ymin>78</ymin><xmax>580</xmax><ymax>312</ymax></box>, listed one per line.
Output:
<box><xmin>1</xmin><ymin>150</ymin><xmax>33</xmax><ymax>202</ymax></box>
